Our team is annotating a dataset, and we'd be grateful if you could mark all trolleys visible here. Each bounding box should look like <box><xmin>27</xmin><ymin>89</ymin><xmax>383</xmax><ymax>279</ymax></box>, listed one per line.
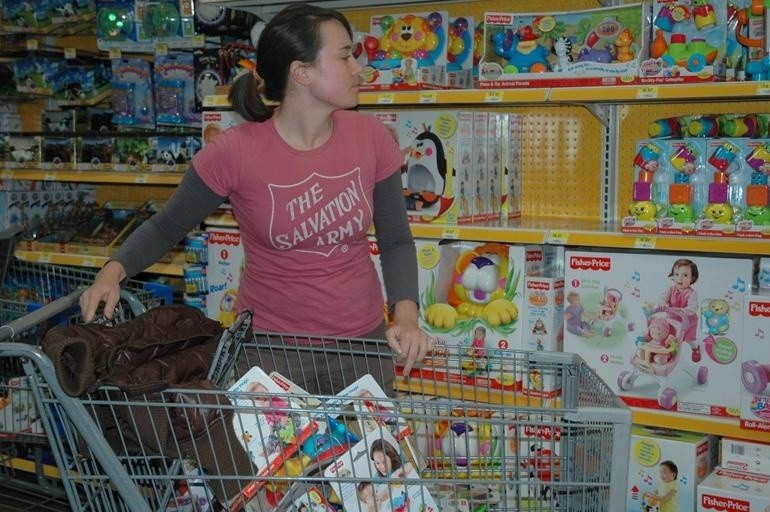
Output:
<box><xmin>0</xmin><ymin>288</ymin><xmax>634</xmax><ymax>512</ymax></box>
<box><xmin>0</xmin><ymin>226</ymin><xmax>173</xmax><ymax>501</ymax></box>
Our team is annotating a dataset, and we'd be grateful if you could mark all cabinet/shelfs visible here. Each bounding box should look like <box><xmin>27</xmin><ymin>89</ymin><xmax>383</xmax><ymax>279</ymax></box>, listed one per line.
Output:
<box><xmin>0</xmin><ymin>0</ymin><xmax>770</xmax><ymax>510</ymax></box>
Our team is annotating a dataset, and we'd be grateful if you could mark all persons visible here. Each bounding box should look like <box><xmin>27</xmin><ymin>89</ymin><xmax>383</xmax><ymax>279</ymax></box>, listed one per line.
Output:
<box><xmin>246</xmin><ymin>380</ymin><xmax>294</xmax><ymax>458</ymax></box>
<box><xmin>79</xmin><ymin>2</ymin><xmax>434</xmax><ymax>397</ymax></box>
<box><xmin>650</xmin><ymin>460</ymin><xmax>680</xmax><ymax>512</ymax></box>
<box><xmin>357</xmin><ymin>438</ymin><xmax>408</xmax><ymax>512</ymax></box>
<box><xmin>565</xmin><ymin>292</ymin><xmax>597</xmax><ymax>338</ymax></box>
<box><xmin>657</xmin><ymin>259</ymin><xmax>702</xmax><ymax>362</ymax></box>
<box><xmin>471</xmin><ymin>327</ymin><xmax>486</xmax><ymax>357</ymax></box>
